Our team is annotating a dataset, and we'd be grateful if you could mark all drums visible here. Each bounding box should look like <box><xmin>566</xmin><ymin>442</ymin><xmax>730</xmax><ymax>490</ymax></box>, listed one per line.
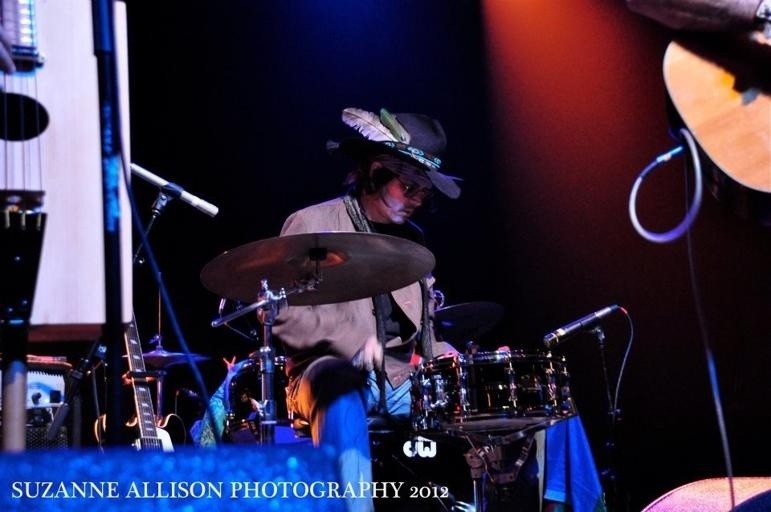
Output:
<box><xmin>410</xmin><ymin>352</ymin><xmax>576</xmax><ymax>434</ymax></box>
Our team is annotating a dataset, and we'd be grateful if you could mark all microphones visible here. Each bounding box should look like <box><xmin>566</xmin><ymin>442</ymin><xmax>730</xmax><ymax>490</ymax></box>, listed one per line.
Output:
<box><xmin>127</xmin><ymin>157</ymin><xmax>226</xmax><ymax>221</ymax></box>
<box><xmin>544</xmin><ymin>305</ymin><xmax>620</xmax><ymax>346</ymax></box>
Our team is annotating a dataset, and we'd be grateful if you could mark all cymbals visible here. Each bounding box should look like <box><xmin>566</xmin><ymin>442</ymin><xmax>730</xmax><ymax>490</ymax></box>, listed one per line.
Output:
<box><xmin>435</xmin><ymin>302</ymin><xmax>503</xmax><ymax>326</ymax></box>
<box><xmin>200</xmin><ymin>232</ymin><xmax>435</xmax><ymax>305</ymax></box>
<box><xmin>121</xmin><ymin>349</ymin><xmax>204</xmax><ymax>367</ymax></box>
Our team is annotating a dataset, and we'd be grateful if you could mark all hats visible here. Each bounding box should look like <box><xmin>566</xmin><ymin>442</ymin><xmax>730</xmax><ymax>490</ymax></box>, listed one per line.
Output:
<box><xmin>374</xmin><ymin>112</ymin><xmax>477</xmax><ymax>200</ymax></box>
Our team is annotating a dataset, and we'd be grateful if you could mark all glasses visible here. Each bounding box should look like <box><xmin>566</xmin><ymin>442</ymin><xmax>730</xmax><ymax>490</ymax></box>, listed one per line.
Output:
<box><xmin>396</xmin><ymin>174</ymin><xmax>437</xmax><ymax>203</ymax></box>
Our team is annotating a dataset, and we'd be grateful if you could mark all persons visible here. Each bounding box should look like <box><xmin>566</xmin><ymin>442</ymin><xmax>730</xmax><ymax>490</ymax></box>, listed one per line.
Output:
<box><xmin>269</xmin><ymin>107</ymin><xmax>463</xmax><ymax>512</ymax></box>
